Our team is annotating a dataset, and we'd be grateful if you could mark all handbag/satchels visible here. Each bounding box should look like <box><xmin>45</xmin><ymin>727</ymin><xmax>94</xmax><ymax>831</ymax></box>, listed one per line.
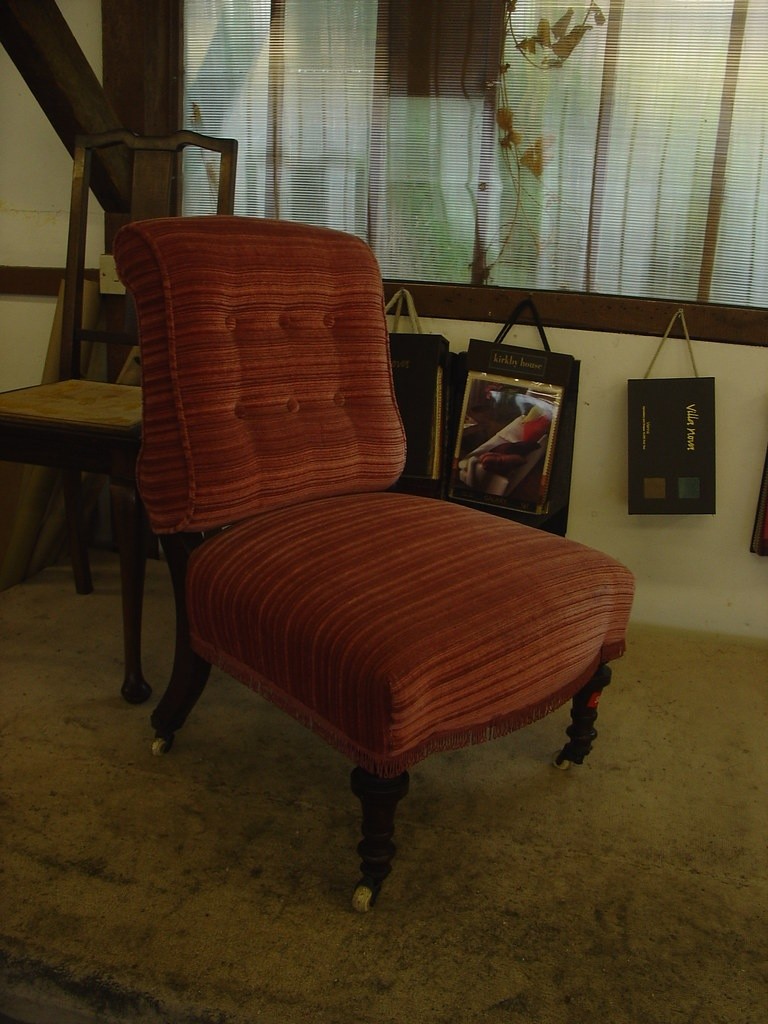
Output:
<box><xmin>750</xmin><ymin>446</ymin><xmax>768</xmax><ymax>557</ymax></box>
<box><xmin>446</xmin><ymin>299</ymin><xmax>581</xmax><ymax>537</ymax></box>
<box><xmin>385</xmin><ymin>288</ymin><xmax>450</xmax><ymax>480</ymax></box>
<box><xmin>627</xmin><ymin>308</ymin><xmax>716</xmax><ymax>514</ymax></box>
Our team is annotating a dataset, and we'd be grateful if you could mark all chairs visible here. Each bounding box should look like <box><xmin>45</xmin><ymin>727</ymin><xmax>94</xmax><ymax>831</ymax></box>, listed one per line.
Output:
<box><xmin>110</xmin><ymin>217</ymin><xmax>635</xmax><ymax>910</ymax></box>
<box><xmin>0</xmin><ymin>130</ymin><xmax>240</xmax><ymax>705</ymax></box>
